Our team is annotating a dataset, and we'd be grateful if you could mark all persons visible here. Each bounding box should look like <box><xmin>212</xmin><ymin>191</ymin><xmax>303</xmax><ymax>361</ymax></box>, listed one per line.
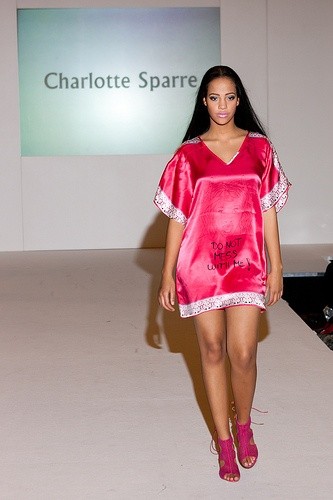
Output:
<box><xmin>154</xmin><ymin>65</ymin><xmax>291</xmax><ymax>481</ymax></box>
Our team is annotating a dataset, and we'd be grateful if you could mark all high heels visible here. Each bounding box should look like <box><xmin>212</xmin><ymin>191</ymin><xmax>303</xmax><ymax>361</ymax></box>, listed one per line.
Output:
<box><xmin>217</xmin><ymin>432</ymin><xmax>241</xmax><ymax>482</ymax></box>
<box><xmin>235</xmin><ymin>415</ymin><xmax>258</xmax><ymax>468</ymax></box>
